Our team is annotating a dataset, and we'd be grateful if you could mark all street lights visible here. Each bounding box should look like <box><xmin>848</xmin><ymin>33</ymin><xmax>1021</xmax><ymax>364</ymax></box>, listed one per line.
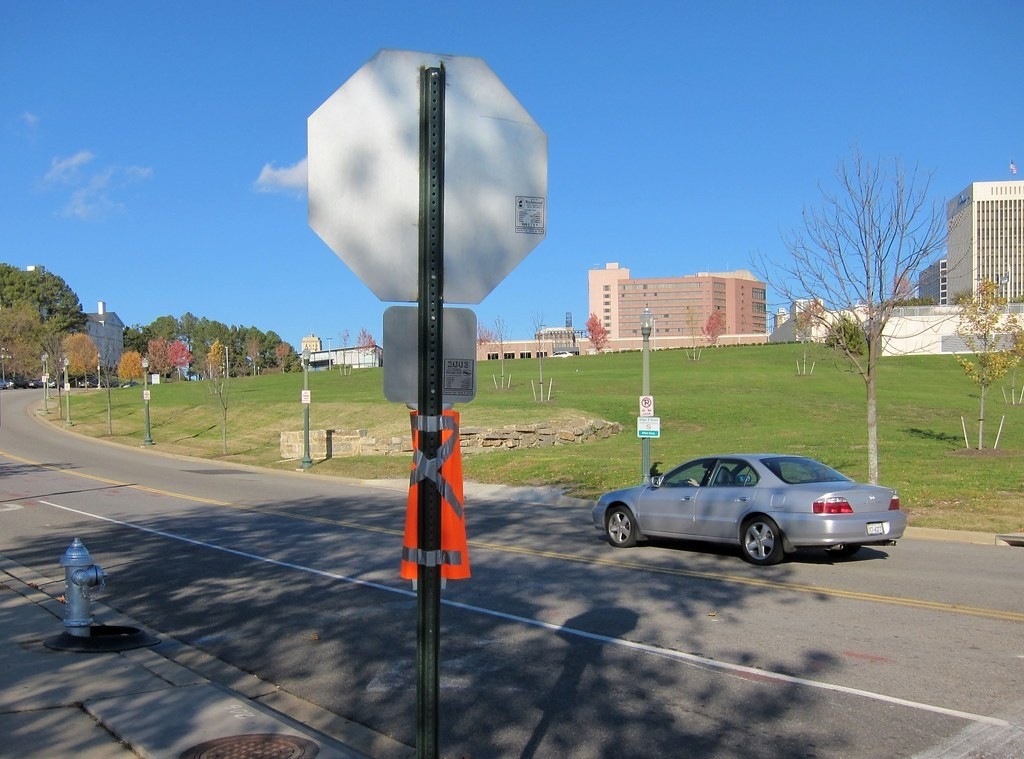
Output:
<box><xmin>97</xmin><ymin>351</ymin><xmax>101</xmax><ymax>389</ymax></box>
<box><xmin>44</xmin><ymin>352</ymin><xmax>49</xmax><ymax>414</ymax></box>
<box><xmin>326</xmin><ymin>337</ymin><xmax>333</xmax><ymax>370</ymax></box>
<box><xmin>638</xmin><ymin>303</ymin><xmax>653</xmax><ymax>486</ymax></box>
<box><xmin>300</xmin><ymin>343</ymin><xmax>313</xmax><ymax>468</ymax></box>
<box><xmin>42</xmin><ymin>355</ymin><xmax>47</xmax><ymax>412</ymax></box>
<box><xmin>540</xmin><ymin>324</ymin><xmax>546</xmax><ymax>356</ymax></box>
<box><xmin>64</xmin><ymin>356</ymin><xmax>74</xmax><ymax>428</ymax></box>
<box><xmin>141</xmin><ymin>357</ymin><xmax>154</xmax><ymax>445</ymax></box>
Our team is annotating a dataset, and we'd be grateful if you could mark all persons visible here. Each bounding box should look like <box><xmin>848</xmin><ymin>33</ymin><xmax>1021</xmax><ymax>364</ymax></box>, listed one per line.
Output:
<box><xmin>688</xmin><ymin>477</ymin><xmax>699</xmax><ymax>487</ymax></box>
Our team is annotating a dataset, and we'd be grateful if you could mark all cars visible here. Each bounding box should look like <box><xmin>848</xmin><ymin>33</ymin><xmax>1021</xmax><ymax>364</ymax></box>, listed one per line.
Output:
<box><xmin>592</xmin><ymin>452</ymin><xmax>906</xmax><ymax>567</ymax></box>
<box><xmin>29</xmin><ymin>378</ymin><xmax>58</xmax><ymax>388</ymax></box>
<box><xmin>0</xmin><ymin>379</ymin><xmax>9</xmax><ymax>389</ymax></box>
<box><xmin>79</xmin><ymin>378</ymin><xmax>99</xmax><ymax>388</ymax></box>
<box><xmin>103</xmin><ymin>380</ymin><xmax>139</xmax><ymax>388</ymax></box>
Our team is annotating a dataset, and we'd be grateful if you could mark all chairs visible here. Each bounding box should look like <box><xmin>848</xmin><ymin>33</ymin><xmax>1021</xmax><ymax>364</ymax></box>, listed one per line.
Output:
<box><xmin>713</xmin><ymin>467</ymin><xmax>736</xmax><ymax>486</ymax></box>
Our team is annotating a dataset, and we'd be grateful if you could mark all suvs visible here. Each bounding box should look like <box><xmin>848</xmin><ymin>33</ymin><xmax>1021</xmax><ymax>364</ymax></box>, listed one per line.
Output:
<box><xmin>12</xmin><ymin>376</ymin><xmax>29</xmax><ymax>389</ymax></box>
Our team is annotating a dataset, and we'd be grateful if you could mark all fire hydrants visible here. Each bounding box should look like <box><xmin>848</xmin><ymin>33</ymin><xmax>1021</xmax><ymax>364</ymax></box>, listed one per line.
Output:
<box><xmin>60</xmin><ymin>538</ymin><xmax>108</xmax><ymax>638</ymax></box>
